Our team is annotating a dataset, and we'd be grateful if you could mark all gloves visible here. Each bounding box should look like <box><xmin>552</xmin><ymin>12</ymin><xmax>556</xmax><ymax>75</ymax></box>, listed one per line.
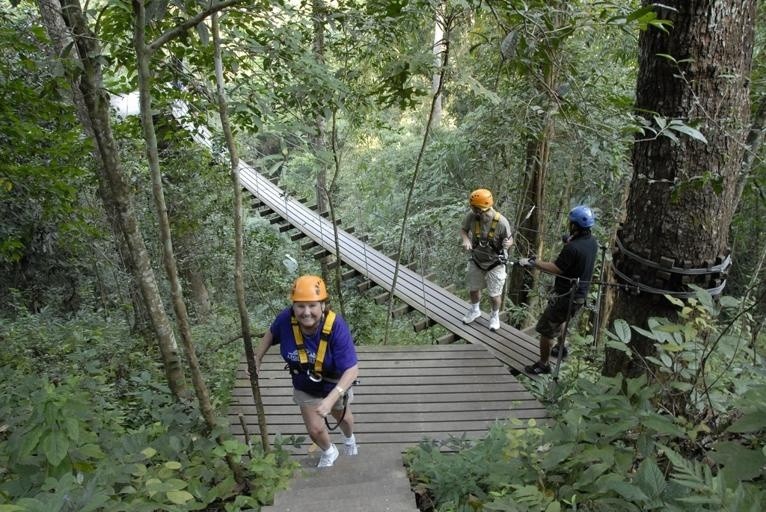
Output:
<box><xmin>461</xmin><ymin>238</ymin><xmax>473</xmax><ymax>250</ymax></box>
<box><xmin>501</xmin><ymin>237</ymin><xmax>513</xmax><ymax>251</ymax></box>
<box><xmin>517</xmin><ymin>256</ymin><xmax>539</xmax><ymax>271</ymax></box>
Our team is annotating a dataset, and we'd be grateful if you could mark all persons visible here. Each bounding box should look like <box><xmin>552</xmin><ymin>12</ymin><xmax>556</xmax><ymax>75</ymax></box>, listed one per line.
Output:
<box><xmin>460</xmin><ymin>189</ymin><xmax>513</xmax><ymax>331</ymax></box>
<box><xmin>245</xmin><ymin>275</ymin><xmax>360</xmax><ymax>470</ymax></box>
<box><xmin>519</xmin><ymin>206</ymin><xmax>598</xmax><ymax>375</ymax></box>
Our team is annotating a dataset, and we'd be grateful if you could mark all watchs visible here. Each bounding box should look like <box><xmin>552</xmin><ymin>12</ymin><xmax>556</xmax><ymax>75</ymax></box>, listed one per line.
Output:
<box><xmin>335</xmin><ymin>385</ymin><xmax>345</xmax><ymax>396</ymax></box>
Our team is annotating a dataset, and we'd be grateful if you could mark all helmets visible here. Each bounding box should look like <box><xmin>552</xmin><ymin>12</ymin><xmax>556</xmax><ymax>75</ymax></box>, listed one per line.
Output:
<box><xmin>567</xmin><ymin>204</ymin><xmax>595</xmax><ymax>230</ymax></box>
<box><xmin>289</xmin><ymin>275</ymin><xmax>329</xmax><ymax>305</ymax></box>
<box><xmin>467</xmin><ymin>189</ymin><xmax>495</xmax><ymax>210</ymax></box>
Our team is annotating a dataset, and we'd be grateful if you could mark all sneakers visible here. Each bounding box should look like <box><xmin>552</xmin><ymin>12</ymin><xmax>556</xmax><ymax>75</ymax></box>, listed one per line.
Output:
<box><xmin>343</xmin><ymin>433</ymin><xmax>358</xmax><ymax>458</ymax></box>
<box><xmin>317</xmin><ymin>441</ymin><xmax>340</xmax><ymax>469</ymax></box>
<box><xmin>462</xmin><ymin>308</ymin><xmax>483</xmax><ymax>324</ymax></box>
<box><xmin>551</xmin><ymin>344</ymin><xmax>569</xmax><ymax>358</ymax></box>
<box><xmin>522</xmin><ymin>358</ymin><xmax>553</xmax><ymax>376</ymax></box>
<box><xmin>487</xmin><ymin>310</ymin><xmax>502</xmax><ymax>332</ymax></box>
